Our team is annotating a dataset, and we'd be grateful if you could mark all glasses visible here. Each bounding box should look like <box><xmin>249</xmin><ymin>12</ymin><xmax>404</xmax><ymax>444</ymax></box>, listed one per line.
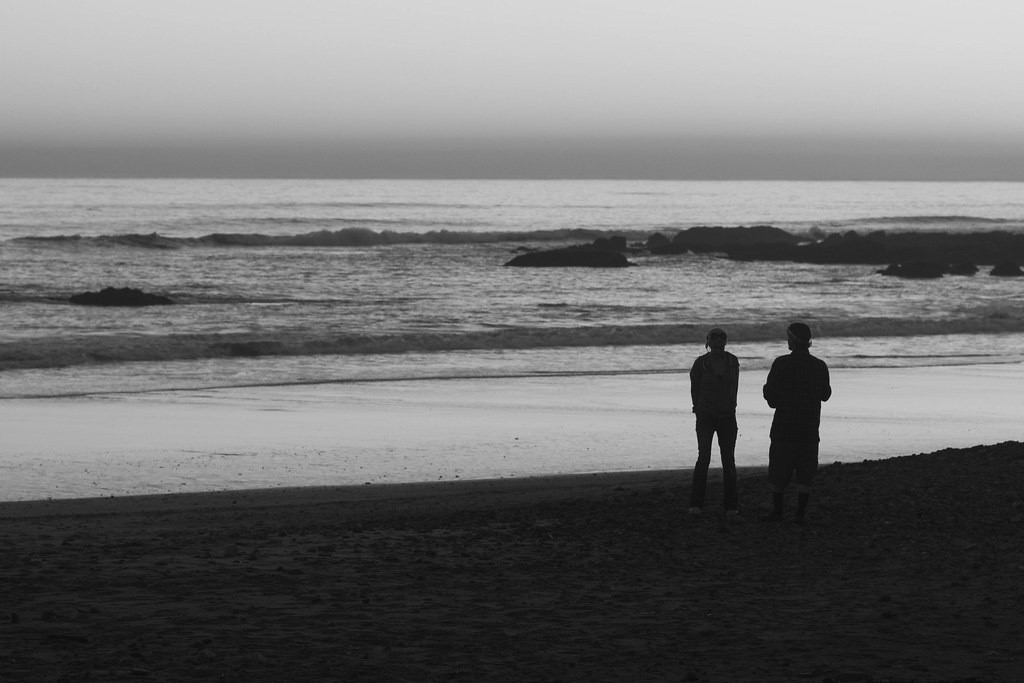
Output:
<box><xmin>710</xmin><ymin>332</ymin><xmax>726</xmax><ymax>341</ymax></box>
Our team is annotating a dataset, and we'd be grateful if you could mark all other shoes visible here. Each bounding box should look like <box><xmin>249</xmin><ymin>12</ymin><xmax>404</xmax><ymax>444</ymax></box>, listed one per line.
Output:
<box><xmin>727</xmin><ymin>509</ymin><xmax>738</xmax><ymax>516</ymax></box>
<box><xmin>688</xmin><ymin>505</ymin><xmax>700</xmax><ymax>515</ymax></box>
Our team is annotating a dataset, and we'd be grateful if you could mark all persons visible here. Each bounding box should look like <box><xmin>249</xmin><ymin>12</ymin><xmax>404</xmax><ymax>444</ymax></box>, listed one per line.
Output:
<box><xmin>688</xmin><ymin>329</ymin><xmax>740</xmax><ymax>515</ymax></box>
<box><xmin>763</xmin><ymin>323</ymin><xmax>831</xmax><ymax>525</ymax></box>
